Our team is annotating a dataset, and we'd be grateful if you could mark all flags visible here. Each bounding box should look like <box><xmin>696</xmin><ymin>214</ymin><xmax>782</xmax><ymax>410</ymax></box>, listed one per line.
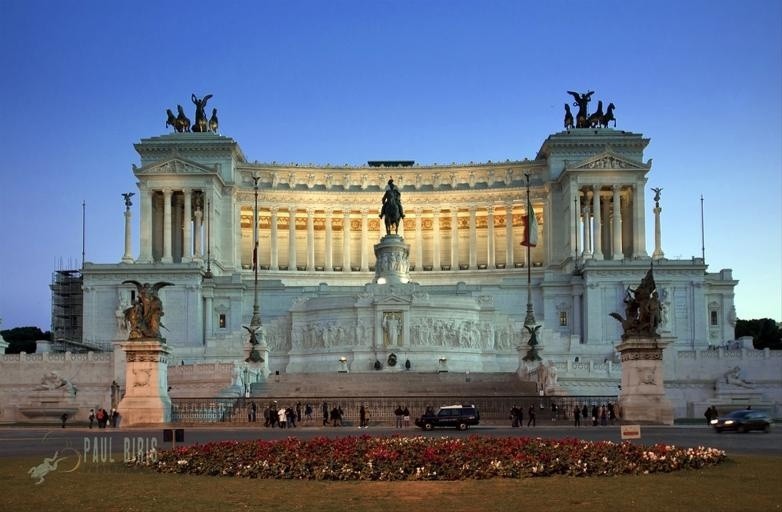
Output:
<box><xmin>520</xmin><ymin>204</ymin><xmax>539</xmax><ymax>247</ymax></box>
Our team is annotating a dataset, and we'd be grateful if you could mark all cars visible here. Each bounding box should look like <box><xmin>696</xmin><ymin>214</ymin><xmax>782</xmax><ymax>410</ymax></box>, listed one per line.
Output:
<box><xmin>710</xmin><ymin>410</ymin><xmax>771</xmax><ymax>432</ymax></box>
<box><xmin>416</xmin><ymin>404</ymin><xmax>480</xmax><ymax>430</ymax></box>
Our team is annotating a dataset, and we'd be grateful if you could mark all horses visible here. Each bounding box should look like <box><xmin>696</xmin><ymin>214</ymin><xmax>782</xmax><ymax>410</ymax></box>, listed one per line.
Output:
<box><xmin>166</xmin><ymin>110</ymin><xmax>183</xmax><ymax>133</ymax></box>
<box><xmin>576</xmin><ymin>102</ymin><xmax>587</xmax><ymax>128</ymax></box>
<box><xmin>384</xmin><ymin>189</ymin><xmax>400</xmax><ymax>235</ymax></box>
<box><xmin>196</xmin><ymin>104</ymin><xmax>208</xmax><ymax>133</ymax></box>
<box><xmin>178</xmin><ymin>105</ymin><xmax>190</xmax><ymax>132</ymax></box>
<box><xmin>601</xmin><ymin>103</ymin><xmax>616</xmax><ymax>128</ymax></box>
<box><xmin>564</xmin><ymin>104</ymin><xmax>575</xmax><ymax>130</ymax></box>
<box><xmin>590</xmin><ymin>101</ymin><xmax>603</xmax><ymax>128</ymax></box>
<box><xmin>209</xmin><ymin>108</ymin><xmax>218</xmax><ymax>133</ymax></box>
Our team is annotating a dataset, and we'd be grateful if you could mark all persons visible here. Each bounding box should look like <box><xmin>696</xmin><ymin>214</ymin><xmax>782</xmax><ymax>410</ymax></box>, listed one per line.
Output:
<box><xmin>60</xmin><ymin>413</ymin><xmax>68</xmax><ymax>428</ymax></box>
<box><xmin>141</xmin><ymin>283</ymin><xmax>153</xmax><ymax>316</ymax></box>
<box><xmin>304</xmin><ymin>320</ymin><xmax>373</xmax><ymax>348</ymax></box>
<box><xmin>425</xmin><ymin>405</ymin><xmax>435</xmax><ymax>416</ymax></box>
<box><xmin>704</xmin><ymin>406</ymin><xmax>718</xmax><ymax>425</ymax></box>
<box><xmin>88</xmin><ymin>408</ymin><xmax>116</xmax><ymax>429</ymax></box>
<box><xmin>410</xmin><ymin>317</ymin><xmax>481</xmax><ymax>349</ymax></box>
<box><xmin>623</xmin><ymin>278</ymin><xmax>662</xmax><ymax>332</ymax></box>
<box><xmin>511</xmin><ymin>401</ymin><xmax>615</xmax><ymax>427</ymax></box>
<box><xmin>746</xmin><ymin>405</ymin><xmax>752</xmax><ymax>410</ymax></box>
<box><xmin>247</xmin><ymin>401</ymin><xmax>370</xmax><ymax>429</ymax></box>
<box><xmin>395</xmin><ymin>406</ymin><xmax>409</xmax><ymax>428</ymax></box>
<box><xmin>379</xmin><ymin>183</ymin><xmax>405</xmax><ymax>219</ymax></box>
<box><xmin>383</xmin><ymin>315</ymin><xmax>401</xmax><ymax>344</ymax></box>
